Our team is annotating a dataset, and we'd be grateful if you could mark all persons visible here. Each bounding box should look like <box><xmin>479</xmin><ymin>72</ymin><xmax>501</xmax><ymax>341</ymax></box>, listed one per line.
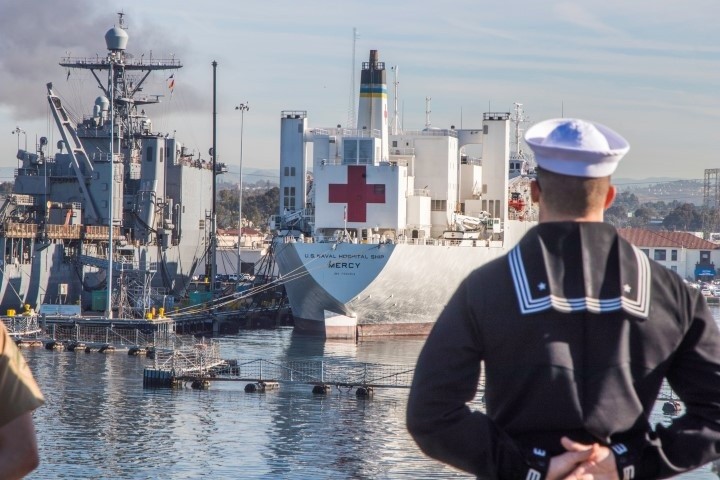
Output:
<box><xmin>0</xmin><ymin>316</ymin><xmax>46</xmax><ymax>480</ymax></box>
<box><xmin>406</xmin><ymin>118</ymin><xmax>720</xmax><ymax>480</ymax></box>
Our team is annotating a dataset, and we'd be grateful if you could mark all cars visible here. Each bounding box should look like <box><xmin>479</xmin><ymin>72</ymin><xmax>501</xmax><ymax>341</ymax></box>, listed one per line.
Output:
<box><xmin>689</xmin><ymin>278</ymin><xmax>720</xmax><ymax>296</ymax></box>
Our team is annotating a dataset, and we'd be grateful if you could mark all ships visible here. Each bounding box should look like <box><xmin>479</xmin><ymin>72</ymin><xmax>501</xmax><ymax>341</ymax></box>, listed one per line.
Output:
<box><xmin>0</xmin><ymin>8</ymin><xmax>292</xmax><ymax>342</ymax></box>
<box><xmin>273</xmin><ymin>49</ymin><xmax>540</xmax><ymax>341</ymax></box>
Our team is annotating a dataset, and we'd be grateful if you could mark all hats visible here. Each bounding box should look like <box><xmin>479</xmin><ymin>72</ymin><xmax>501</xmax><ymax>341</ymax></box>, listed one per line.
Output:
<box><xmin>524</xmin><ymin>118</ymin><xmax>630</xmax><ymax>178</ymax></box>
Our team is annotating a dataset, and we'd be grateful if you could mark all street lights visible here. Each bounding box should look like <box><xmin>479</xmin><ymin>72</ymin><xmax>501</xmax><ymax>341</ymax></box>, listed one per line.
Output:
<box><xmin>233</xmin><ymin>102</ymin><xmax>249</xmax><ymax>275</ymax></box>
<box><xmin>12</xmin><ymin>127</ymin><xmax>25</xmax><ymax>171</ymax></box>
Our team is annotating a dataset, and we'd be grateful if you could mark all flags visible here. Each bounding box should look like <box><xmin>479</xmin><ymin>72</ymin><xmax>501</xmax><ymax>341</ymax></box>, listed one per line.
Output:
<box><xmin>166</xmin><ymin>72</ymin><xmax>175</xmax><ymax>89</ymax></box>
